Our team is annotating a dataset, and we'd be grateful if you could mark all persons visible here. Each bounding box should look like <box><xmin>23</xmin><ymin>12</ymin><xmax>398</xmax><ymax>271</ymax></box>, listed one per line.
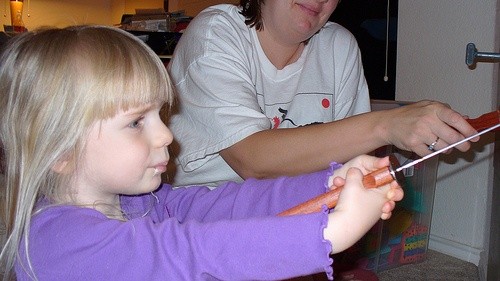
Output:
<box><xmin>164</xmin><ymin>1</ymin><xmax>480</xmax><ymax>190</ymax></box>
<box><xmin>0</xmin><ymin>22</ymin><xmax>406</xmax><ymax>280</ymax></box>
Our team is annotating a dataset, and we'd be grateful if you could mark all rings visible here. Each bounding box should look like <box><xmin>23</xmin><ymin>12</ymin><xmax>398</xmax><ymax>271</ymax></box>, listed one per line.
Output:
<box><xmin>428</xmin><ymin>136</ymin><xmax>440</xmax><ymax>150</ymax></box>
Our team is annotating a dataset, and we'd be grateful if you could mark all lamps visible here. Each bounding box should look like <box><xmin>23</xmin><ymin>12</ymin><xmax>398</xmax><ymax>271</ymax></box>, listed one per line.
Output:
<box><xmin>3</xmin><ymin>0</ymin><xmax>31</xmax><ymax>34</ymax></box>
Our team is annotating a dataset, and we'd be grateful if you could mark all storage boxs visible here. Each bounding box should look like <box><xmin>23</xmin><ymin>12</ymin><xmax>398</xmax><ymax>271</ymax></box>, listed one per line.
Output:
<box><xmin>350</xmin><ymin>96</ymin><xmax>443</xmax><ymax>273</ymax></box>
